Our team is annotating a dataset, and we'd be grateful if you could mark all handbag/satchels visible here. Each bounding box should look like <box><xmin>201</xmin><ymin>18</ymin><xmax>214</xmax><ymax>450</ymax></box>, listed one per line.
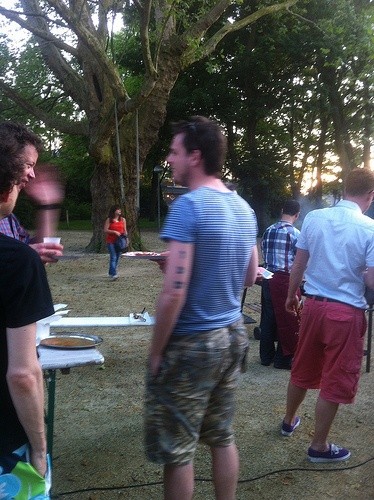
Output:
<box><xmin>115</xmin><ymin>234</ymin><xmax>128</xmax><ymax>252</ymax></box>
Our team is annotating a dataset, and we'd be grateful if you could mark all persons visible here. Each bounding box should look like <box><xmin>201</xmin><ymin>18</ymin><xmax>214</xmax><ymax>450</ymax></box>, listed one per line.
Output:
<box><xmin>0</xmin><ymin>124</ymin><xmax>57</xmax><ymax>500</ymax></box>
<box><xmin>0</xmin><ymin>119</ymin><xmax>62</xmax><ymax>266</ymax></box>
<box><xmin>259</xmin><ymin>199</ymin><xmax>301</xmax><ymax>370</ymax></box>
<box><xmin>141</xmin><ymin>116</ymin><xmax>258</xmax><ymax>500</ymax></box>
<box><xmin>280</xmin><ymin>167</ymin><xmax>374</xmax><ymax>463</ymax></box>
<box><xmin>104</xmin><ymin>204</ymin><xmax>130</xmax><ymax>281</ymax></box>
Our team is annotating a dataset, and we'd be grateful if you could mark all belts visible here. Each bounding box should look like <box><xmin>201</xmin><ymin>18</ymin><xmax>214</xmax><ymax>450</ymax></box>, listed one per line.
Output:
<box><xmin>306</xmin><ymin>294</ymin><xmax>339</xmax><ymax>302</ymax></box>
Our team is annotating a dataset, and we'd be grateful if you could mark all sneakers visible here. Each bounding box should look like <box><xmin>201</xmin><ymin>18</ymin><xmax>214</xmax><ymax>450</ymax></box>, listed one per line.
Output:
<box><xmin>280</xmin><ymin>416</ymin><xmax>301</xmax><ymax>434</ymax></box>
<box><xmin>306</xmin><ymin>441</ymin><xmax>350</xmax><ymax>463</ymax></box>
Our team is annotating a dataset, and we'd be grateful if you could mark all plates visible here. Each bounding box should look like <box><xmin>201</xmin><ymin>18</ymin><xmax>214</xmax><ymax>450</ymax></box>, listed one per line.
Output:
<box><xmin>40</xmin><ymin>332</ymin><xmax>103</xmax><ymax>348</ymax></box>
<box><xmin>121</xmin><ymin>252</ymin><xmax>170</xmax><ymax>259</ymax></box>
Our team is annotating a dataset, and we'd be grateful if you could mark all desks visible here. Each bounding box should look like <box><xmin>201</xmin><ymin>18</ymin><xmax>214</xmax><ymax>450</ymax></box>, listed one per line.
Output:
<box><xmin>32</xmin><ymin>334</ymin><xmax>106</xmax><ymax>468</ymax></box>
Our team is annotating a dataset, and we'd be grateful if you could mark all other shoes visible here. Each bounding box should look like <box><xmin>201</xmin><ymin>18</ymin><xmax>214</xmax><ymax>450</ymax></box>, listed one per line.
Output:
<box><xmin>111</xmin><ymin>275</ymin><xmax>119</xmax><ymax>281</ymax></box>
<box><xmin>260</xmin><ymin>352</ymin><xmax>273</xmax><ymax>366</ymax></box>
<box><xmin>272</xmin><ymin>356</ymin><xmax>291</xmax><ymax>370</ymax></box>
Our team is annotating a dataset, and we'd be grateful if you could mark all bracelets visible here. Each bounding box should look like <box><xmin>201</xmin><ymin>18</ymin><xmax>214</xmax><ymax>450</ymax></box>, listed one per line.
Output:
<box><xmin>35</xmin><ymin>203</ymin><xmax>60</xmax><ymax>211</ymax></box>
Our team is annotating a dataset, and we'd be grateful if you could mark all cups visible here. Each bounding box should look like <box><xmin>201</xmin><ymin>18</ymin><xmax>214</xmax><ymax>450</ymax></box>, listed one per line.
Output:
<box><xmin>43</xmin><ymin>238</ymin><xmax>61</xmax><ymax>245</ymax></box>
